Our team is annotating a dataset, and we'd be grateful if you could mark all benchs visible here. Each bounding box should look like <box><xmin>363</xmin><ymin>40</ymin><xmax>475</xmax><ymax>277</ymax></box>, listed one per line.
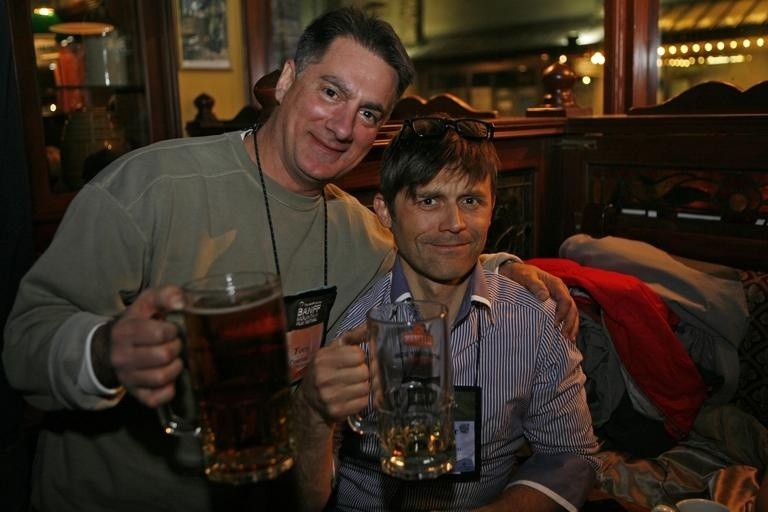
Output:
<box><xmin>561</xmin><ymin>266</ymin><xmax>768</xmax><ymax>512</ymax></box>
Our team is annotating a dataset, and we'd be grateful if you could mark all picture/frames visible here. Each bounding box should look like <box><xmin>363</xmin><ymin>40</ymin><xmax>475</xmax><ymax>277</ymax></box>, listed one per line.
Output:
<box><xmin>172</xmin><ymin>0</ymin><xmax>232</xmax><ymax>71</ymax></box>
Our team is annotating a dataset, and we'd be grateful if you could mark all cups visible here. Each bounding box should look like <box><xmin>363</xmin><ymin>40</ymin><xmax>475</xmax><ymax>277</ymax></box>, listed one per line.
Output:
<box><xmin>156</xmin><ymin>272</ymin><xmax>298</xmax><ymax>482</ymax></box>
<box><xmin>345</xmin><ymin>298</ymin><xmax>457</xmax><ymax>478</ymax></box>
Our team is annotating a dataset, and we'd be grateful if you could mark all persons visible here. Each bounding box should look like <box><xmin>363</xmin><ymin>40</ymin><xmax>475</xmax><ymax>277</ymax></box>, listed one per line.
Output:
<box><xmin>1</xmin><ymin>3</ymin><xmax>581</xmax><ymax>512</ymax></box>
<box><xmin>284</xmin><ymin>112</ymin><xmax>603</xmax><ymax>512</ymax></box>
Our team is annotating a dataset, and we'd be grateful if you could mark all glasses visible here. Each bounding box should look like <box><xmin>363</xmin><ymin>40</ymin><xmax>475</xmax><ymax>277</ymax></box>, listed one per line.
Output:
<box><xmin>397</xmin><ymin>114</ymin><xmax>497</xmax><ymax>145</ymax></box>
